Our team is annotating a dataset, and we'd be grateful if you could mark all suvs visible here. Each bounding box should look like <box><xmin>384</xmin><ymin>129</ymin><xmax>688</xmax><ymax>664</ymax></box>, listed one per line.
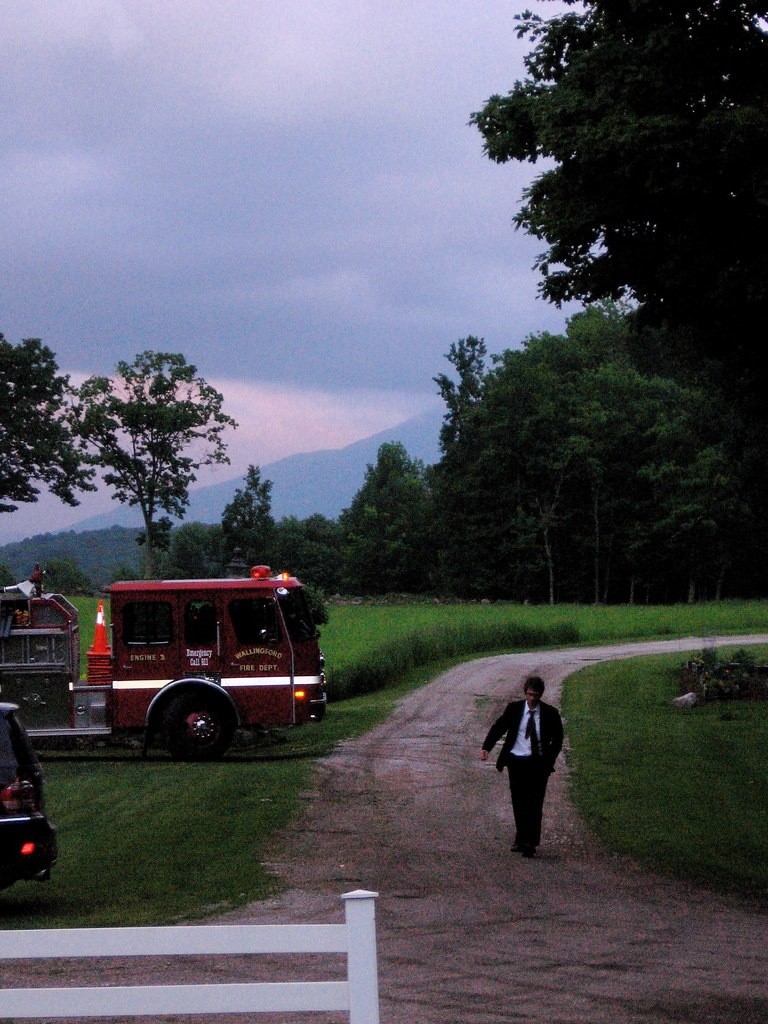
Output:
<box><xmin>0</xmin><ymin>702</ymin><xmax>57</xmax><ymax>888</ymax></box>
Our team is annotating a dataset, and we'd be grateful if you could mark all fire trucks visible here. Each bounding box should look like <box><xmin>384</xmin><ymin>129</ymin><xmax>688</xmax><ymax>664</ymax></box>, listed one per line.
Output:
<box><xmin>0</xmin><ymin>564</ymin><xmax>327</xmax><ymax>761</ymax></box>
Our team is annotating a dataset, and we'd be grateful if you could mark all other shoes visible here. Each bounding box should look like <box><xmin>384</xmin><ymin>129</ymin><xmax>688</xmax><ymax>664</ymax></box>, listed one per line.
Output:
<box><xmin>511</xmin><ymin>841</ymin><xmax>523</xmax><ymax>852</ymax></box>
<box><xmin>522</xmin><ymin>844</ymin><xmax>534</xmax><ymax>858</ymax></box>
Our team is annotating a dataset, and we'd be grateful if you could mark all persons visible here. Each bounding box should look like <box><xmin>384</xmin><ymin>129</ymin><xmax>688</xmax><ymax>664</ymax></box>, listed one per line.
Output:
<box><xmin>479</xmin><ymin>677</ymin><xmax>564</xmax><ymax>858</ymax></box>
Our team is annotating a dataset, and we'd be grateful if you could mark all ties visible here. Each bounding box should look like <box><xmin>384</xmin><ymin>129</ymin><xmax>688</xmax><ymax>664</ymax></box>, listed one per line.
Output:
<box><xmin>525</xmin><ymin>710</ymin><xmax>540</xmax><ymax>760</ymax></box>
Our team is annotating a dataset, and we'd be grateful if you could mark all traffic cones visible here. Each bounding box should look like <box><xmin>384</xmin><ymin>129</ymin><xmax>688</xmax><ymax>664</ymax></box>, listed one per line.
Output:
<box><xmin>85</xmin><ymin>599</ymin><xmax>112</xmax><ymax>685</ymax></box>
